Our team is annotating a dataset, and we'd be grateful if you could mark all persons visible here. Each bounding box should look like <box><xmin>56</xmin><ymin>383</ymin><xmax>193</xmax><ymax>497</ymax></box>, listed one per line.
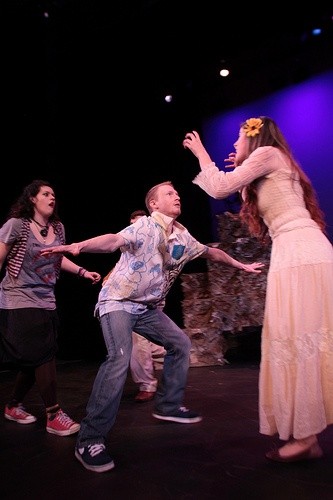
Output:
<box><xmin>183</xmin><ymin>116</ymin><xmax>332</xmax><ymax>459</ymax></box>
<box><xmin>40</xmin><ymin>182</ymin><xmax>266</xmax><ymax>473</ymax></box>
<box><xmin>101</xmin><ymin>209</ymin><xmax>166</xmax><ymax>401</ymax></box>
<box><xmin>0</xmin><ymin>181</ymin><xmax>101</xmax><ymax>437</ymax></box>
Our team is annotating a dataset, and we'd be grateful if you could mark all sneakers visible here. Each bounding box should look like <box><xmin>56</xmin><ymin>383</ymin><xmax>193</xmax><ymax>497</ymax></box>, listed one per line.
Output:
<box><xmin>4</xmin><ymin>404</ymin><xmax>36</xmax><ymax>424</ymax></box>
<box><xmin>151</xmin><ymin>403</ymin><xmax>202</xmax><ymax>424</ymax></box>
<box><xmin>46</xmin><ymin>406</ymin><xmax>80</xmax><ymax>436</ymax></box>
<box><xmin>75</xmin><ymin>441</ymin><xmax>114</xmax><ymax>472</ymax></box>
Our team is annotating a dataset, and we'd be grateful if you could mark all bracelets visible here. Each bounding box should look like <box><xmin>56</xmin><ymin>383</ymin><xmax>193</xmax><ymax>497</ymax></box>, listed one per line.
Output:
<box><xmin>76</xmin><ymin>267</ymin><xmax>84</xmax><ymax>274</ymax></box>
<box><xmin>81</xmin><ymin>271</ymin><xmax>88</xmax><ymax>277</ymax></box>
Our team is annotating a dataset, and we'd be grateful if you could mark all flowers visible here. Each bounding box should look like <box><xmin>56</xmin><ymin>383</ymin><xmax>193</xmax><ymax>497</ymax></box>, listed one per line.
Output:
<box><xmin>243</xmin><ymin>117</ymin><xmax>264</xmax><ymax>137</ymax></box>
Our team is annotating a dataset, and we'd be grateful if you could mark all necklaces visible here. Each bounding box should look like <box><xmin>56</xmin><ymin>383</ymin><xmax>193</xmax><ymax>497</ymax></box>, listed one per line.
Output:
<box><xmin>32</xmin><ymin>217</ymin><xmax>50</xmax><ymax>237</ymax></box>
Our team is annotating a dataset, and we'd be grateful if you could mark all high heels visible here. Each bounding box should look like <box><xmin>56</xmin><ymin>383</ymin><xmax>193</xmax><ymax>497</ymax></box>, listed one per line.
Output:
<box><xmin>265</xmin><ymin>438</ymin><xmax>322</xmax><ymax>461</ymax></box>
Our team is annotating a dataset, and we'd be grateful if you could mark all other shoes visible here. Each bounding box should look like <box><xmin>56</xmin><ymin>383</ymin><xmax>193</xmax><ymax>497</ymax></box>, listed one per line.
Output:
<box><xmin>135</xmin><ymin>390</ymin><xmax>156</xmax><ymax>402</ymax></box>
